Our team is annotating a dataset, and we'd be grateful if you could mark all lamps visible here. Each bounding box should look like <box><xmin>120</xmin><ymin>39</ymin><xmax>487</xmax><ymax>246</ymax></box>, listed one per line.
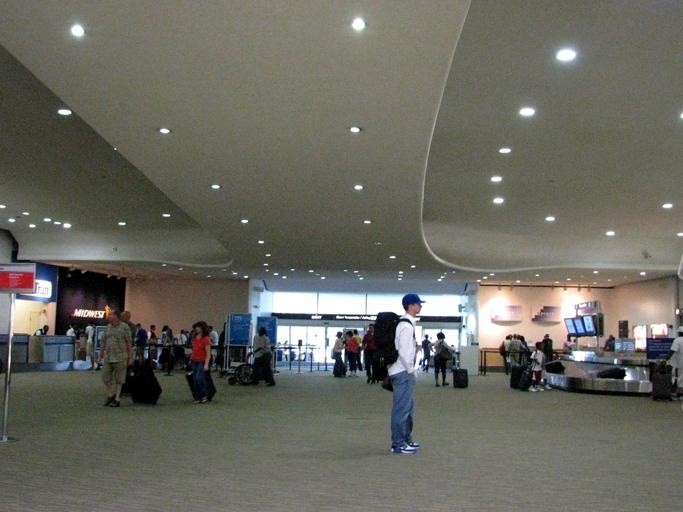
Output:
<box><xmin>480</xmin><ymin>279</ymin><xmax>616</xmax><ymax>293</ymax></box>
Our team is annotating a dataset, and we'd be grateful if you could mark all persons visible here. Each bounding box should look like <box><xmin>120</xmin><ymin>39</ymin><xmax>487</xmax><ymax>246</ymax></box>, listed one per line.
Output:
<box><xmin>188</xmin><ymin>319</ymin><xmax>210</xmax><ymax>405</ymax></box>
<box><xmin>428</xmin><ymin>332</ymin><xmax>452</xmax><ymax>386</ymax></box>
<box><xmin>664</xmin><ymin>325</ymin><xmax>683</xmax><ymax>401</ymax></box>
<box><xmin>251</xmin><ymin>326</ymin><xmax>276</xmax><ymax>386</ymax></box>
<box><xmin>602</xmin><ymin>334</ymin><xmax>615</xmax><ymax>352</ymax></box>
<box><xmin>129</xmin><ymin>322</ymin><xmax>226</xmax><ymax>373</ymax></box>
<box><xmin>386</xmin><ymin>292</ymin><xmax>426</xmax><ymax>455</ymax></box>
<box><xmin>65</xmin><ymin>321</ymin><xmax>95</xmax><ymax>356</ymax></box>
<box><xmin>562</xmin><ymin>333</ymin><xmax>577</xmax><ymax>350</ymax></box>
<box><xmin>422</xmin><ymin>335</ymin><xmax>433</xmax><ymax>367</ymax></box>
<box><xmin>498</xmin><ymin>332</ymin><xmax>555</xmax><ymax>393</ymax></box>
<box><xmin>333</xmin><ymin>329</ymin><xmax>362</xmax><ymax>377</ymax></box>
<box><xmin>34</xmin><ymin>325</ymin><xmax>49</xmax><ymax>336</ymax></box>
<box><xmin>361</xmin><ymin>324</ymin><xmax>378</xmax><ymax>384</ymax></box>
<box><xmin>96</xmin><ymin>310</ymin><xmax>132</xmax><ymax>407</ymax></box>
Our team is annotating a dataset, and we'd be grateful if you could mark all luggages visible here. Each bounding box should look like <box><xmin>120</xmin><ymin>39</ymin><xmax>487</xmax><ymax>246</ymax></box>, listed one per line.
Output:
<box><xmin>186</xmin><ymin>363</ymin><xmax>200</xmax><ymax>400</ymax></box>
<box><xmin>511</xmin><ymin>350</ymin><xmax>523</xmax><ymax>390</ymax></box>
<box><xmin>652</xmin><ymin>359</ymin><xmax>672</xmax><ymax>401</ymax></box>
<box><xmin>453</xmin><ymin>352</ymin><xmax>468</xmax><ymax>388</ymax></box>
<box><xmin>125</xmin><ymin>361</ymin><xmax>161</xmax><ymax>404</ymax></box>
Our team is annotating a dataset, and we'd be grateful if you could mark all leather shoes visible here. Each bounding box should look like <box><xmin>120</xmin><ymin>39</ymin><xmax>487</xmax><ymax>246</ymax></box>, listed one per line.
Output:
<box><xmin>104</xmin><ymin>395</ymin><xmax>122</xmax><ymax>405</ymax></box>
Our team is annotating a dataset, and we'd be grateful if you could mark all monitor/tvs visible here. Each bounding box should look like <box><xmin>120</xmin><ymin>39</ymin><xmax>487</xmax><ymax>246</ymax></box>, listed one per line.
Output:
<box><xmin>564</xmin><ymin>318</ymin><xmax>578</xmax><ymax>336</ymax></box>
<box><xmin>646</xmin><ymin>338</ymin><xmax>675</xmax><ymax>359</ymax></box>
<box><xmin>582</xmin><ymin>315</ymin><xmax>597</xmax><ymax>336</ymax></box>
<box><xmin>572</xmin><ymin>316</ymin><xmax>586</xmax><ymax>337</ymax></box>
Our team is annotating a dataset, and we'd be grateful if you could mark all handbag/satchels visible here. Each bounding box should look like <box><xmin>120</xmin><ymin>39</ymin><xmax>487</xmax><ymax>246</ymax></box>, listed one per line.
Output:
<box><xmin>545</xmin><ymin>361</ymin><xmax>564</xmax><ymax>373</ymax></box>
<box><xmin>439</xmin><ymin>340</ymin><xmax>450</xmax><ymax>360</ymax></box>
<box><xmin>517</xmin><ymin>369</ymin><xmax>533</xmax><ymax>390</ymax></box>
<box><xmin>381</xmin><ymin>377</ymin><xmax>393</xmax><ymax>392</ymax></box>
<box><xmin>597</xmin><ymin>367</ymin><xmax>626</xmax><ymax>379</ymax></box>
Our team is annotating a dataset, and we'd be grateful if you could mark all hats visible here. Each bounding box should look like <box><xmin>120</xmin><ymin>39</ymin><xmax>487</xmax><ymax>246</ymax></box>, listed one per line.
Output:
<box><xmin>403</xmin><ymin>294</ymin><xmax>426</xmax><ymax>304</ymax></box>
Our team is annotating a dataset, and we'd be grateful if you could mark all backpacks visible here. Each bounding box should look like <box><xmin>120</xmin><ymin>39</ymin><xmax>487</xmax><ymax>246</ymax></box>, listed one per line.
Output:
<box><xmin>373</xmin><ymin>312</ymin><xmax>414</xmax><ymax>364</ymax></box>
